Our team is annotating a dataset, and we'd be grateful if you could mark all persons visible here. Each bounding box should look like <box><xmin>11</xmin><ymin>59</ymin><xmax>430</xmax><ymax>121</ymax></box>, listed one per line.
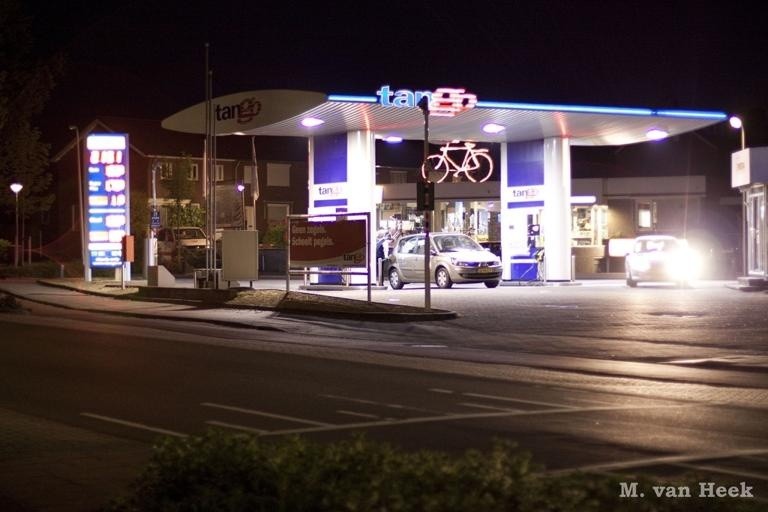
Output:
<box><xmin>376</xmin><ymin>233</ymin><xmax>393</xmax><ymax>286</ymax></box>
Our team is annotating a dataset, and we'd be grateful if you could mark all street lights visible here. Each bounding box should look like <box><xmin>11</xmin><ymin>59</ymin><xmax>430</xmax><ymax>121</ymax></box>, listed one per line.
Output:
<box><xmin>10</xmin><ymin>183</ymin><xmax>23</xmax><ymax>266</ymax></box>
<box><xmin>237</xmin><ymin>179</ymin><xmax>245</xmax><ymax>230</ymax></box>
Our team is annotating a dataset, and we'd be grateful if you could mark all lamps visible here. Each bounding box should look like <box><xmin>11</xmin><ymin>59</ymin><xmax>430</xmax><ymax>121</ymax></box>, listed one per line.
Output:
<box><xmin>726</xmin><ymin>113</ymin><xmax>750</xmax><ymax>149</ymax></box>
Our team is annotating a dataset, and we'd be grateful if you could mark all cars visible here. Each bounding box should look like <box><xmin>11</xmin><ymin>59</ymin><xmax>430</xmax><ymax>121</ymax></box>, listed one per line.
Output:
<box><xmin>156</xmin><ymin>226</ymin><xmax>210</xmax><ymax>272</ymax></box>
<box><xmin>386</xmin><ymin>233</ymin><xmax>502</xmax><ymax>290</ymax></box>
<box><xmin>624</xmin><ymin>236</ymin><xmax>698</xmax><ymax>289</ymax></box>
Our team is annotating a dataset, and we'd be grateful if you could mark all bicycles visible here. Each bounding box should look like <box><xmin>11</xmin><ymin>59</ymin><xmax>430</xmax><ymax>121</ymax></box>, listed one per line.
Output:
<box><xmin>421</xmin><ymin>139</ymin><xmax>493</xmax><ymax>183</ymax></box>
